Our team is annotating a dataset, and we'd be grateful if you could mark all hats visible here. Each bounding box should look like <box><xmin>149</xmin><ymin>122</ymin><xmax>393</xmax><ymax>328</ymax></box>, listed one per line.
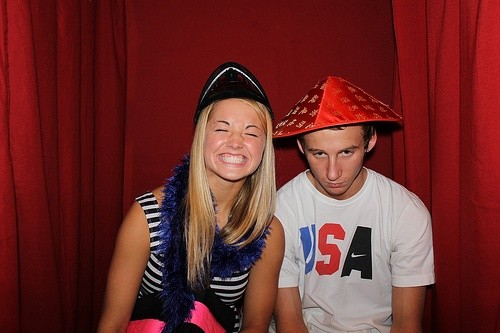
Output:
<box><xmin>194</xmin><ymin>61</ymin><xmax>271</xmax><ymax>122</ymax></box>
<box><xmin>272</xmin><ymin>76</ymin><xmax>401</xmax><ymax>138</ymax></box>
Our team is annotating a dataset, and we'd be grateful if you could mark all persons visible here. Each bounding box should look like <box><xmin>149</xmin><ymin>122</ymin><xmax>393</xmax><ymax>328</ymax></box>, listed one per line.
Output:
<box><xmin>97</xmin><ymin>91</ymin><xmax>286</xmax><ymax>333</ymax></box>
<box><xmin>263</xmin><ymin>108</ymin><xmax>436</xmax><ymax>333</ymax></box>
